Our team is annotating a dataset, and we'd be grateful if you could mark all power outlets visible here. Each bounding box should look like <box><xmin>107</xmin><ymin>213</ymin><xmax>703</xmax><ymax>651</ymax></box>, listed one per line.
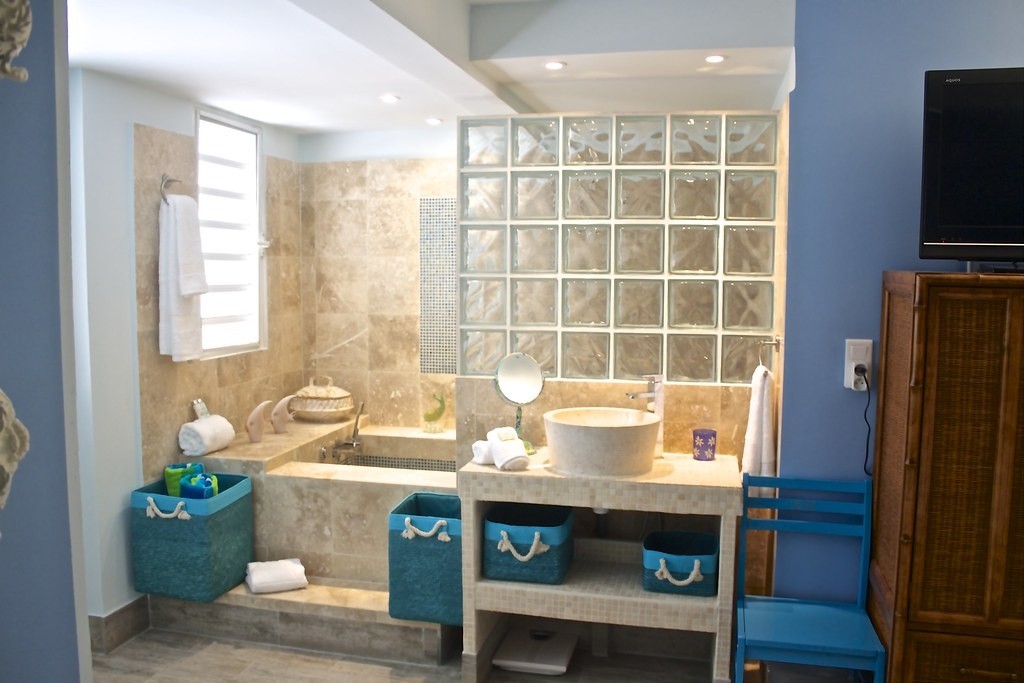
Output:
<box><xmin>844</xmin><ymin>338</ymin><xmax>873</xmax><ymax>392</ymax></box>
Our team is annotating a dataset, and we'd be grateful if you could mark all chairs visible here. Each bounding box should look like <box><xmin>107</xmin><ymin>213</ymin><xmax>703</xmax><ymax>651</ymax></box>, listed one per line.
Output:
<box><xmin>735</xmin><ymin>472</ymin><xmax>887</xmax><ymax>683</ymax></box>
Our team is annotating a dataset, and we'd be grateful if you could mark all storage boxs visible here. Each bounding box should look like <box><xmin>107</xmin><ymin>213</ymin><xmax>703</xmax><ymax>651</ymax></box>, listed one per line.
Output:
<box><xmin>483</xmin><ymin>502</ymin><xmax>576</xmax><ymax>584</ymax></box>
<box><xmin>388</xmin><ymin>491</ymin><xmax>463</xmax><ymax>626</ymax></box>
<box><xmin>643</xmin><ymin>530</ymin><xmax>718</xmax><ymax>595</ymax></box>
<box><xmin>130</xmin><ymin>472</ymin><xmax>254</xmax><ymax>604</ymax></box>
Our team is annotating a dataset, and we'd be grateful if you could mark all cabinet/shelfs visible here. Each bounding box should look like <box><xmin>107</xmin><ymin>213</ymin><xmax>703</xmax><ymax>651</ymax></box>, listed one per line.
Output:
<box><xmin>868</xmin><ymin>272</ymin><xmax>1024</xmax><ymax>683</ymax></box>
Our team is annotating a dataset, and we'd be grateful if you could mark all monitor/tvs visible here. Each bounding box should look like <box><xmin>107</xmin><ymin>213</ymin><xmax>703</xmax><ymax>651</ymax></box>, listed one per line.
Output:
<box><xmin>920</xmin><ymin>65</ymin><xmax>1024</xmax><ymax>273</ymax></box>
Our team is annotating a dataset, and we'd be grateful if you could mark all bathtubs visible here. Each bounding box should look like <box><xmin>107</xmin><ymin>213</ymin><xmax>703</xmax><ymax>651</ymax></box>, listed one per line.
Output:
<box><xmin>266</xmin><ymin>425</ymin><xmax>456</xmax><ymax>583</ymax></box>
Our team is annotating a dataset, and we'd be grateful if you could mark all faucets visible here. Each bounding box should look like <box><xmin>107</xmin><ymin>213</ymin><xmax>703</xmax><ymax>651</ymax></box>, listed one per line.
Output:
<box><xmin>332</xmin><ymin>401</ymin><xmax>365</xmax><ymax>458</ymax></box>
<box><xmin>626</xmin><ymin>374</ymin><xmax>665</xmax><ymax>458</ymax></box>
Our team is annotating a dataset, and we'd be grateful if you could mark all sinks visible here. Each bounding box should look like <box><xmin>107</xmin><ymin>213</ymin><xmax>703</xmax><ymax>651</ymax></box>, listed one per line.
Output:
<box><xmin>543</xmin><ymin>406</ymin><xmax>662</xmax><ymax>478</ymax></box>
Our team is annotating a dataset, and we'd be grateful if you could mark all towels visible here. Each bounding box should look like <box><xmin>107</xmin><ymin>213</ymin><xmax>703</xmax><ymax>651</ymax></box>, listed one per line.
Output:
<box><xmin>740</xmin><ymin>364</ymin><xmax>775</xmax><ymax>499</ymax></box>
<box><xmin>245</xmin><ymin>558</ymin><xmax>309</xmax><ymax>594</ymax></box>
<box><xmin>179</xmin><ymin>414</ymin><xmax>236</xmax><ymax>456</ymax></box>
<box><xmin>471</xmin><ymin>426</ymin><xmax>530</xmax><ymax>471</ymax></box>
<box><xmin>157</xmin><ymin>193</ymin><xmax>209</xmax><ymax>363</ymax></box>
<box><xmin>163</xmin><ymin>462</ymin><xmax>219</xmax><ymax>500</ymax></box>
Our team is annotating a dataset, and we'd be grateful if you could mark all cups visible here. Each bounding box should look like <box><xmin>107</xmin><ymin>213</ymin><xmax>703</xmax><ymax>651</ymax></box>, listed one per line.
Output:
<box><xmin>694</xmin><ymin>429</ymin><xmax>717</xmax><ymax>461</ymax></box>
